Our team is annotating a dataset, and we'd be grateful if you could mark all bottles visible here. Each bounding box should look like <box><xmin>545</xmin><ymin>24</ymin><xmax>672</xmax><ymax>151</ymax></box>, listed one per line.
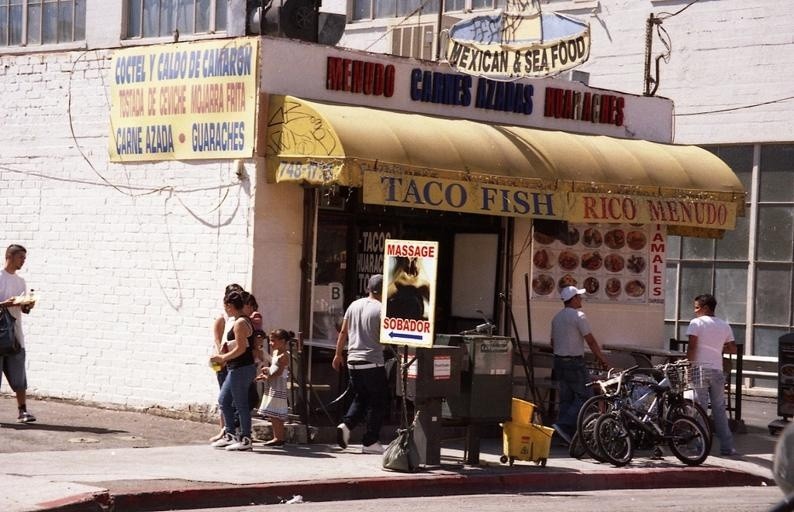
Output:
<box><xmin>23</xmin><ymin>287</ymin><xmax>34</xmax><ymax>314</ymax></box>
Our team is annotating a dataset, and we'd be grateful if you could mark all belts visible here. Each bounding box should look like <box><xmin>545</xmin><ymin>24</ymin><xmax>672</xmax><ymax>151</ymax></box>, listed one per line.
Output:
<box><xmin>346</xmin><ymin>362</ymin><xmax>384</xmax><ymax>369</ymax></box>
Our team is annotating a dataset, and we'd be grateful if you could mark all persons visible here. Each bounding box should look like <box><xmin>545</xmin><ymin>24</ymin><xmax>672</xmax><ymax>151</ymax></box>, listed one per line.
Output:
<box><xmin>551</xmin><ymin>284</ymin><xmax>610</xmax><ymax>449</ymax></box>
<box><xmin>687</xmin><ymin>295</ymin><xmax>740</xmax><ymax>459</ymax></box>
<box><xmin>253</xmin><ymin>327</ymin><xmax>295</xmax><ymax>448</ymax></box>
<box><xmin>208</xmin><ymin>277</ymin><xmax>268</xmax><ymax>449</ymax></box>
<box><xmin>330</xmin><ymin>274</ymin><xmax>406</xmax><ymax>456</ymax></box>
<box><xmin>1</xmin><ymin>243</ymin><xmax>39</xmax><ymax>426</ymax></box>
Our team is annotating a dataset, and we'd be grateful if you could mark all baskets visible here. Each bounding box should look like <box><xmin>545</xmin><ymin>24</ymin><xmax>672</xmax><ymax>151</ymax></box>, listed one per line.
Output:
<box><xmin>670</xmin><ymin>361</ymin><xmax>713</xmax><ymax>389</ymax></box>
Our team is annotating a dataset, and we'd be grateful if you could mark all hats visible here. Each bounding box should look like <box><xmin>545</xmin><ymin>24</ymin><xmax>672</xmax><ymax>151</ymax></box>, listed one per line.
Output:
<box><xmin>368</xmin><ymin>274</ymin><xmax>382</xmax><ymax>294</ymax></box>
<box><xmin>560</xmin><ymin>286</ymin><xmax>585</xmax><ymax>302</ymax></box>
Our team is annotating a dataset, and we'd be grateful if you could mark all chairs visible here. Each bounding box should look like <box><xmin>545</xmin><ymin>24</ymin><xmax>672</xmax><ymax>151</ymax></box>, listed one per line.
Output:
<box><xmin>513</xmin><ymin>352</ymin><xmax>558</xmax><ymax>409</ymax></box>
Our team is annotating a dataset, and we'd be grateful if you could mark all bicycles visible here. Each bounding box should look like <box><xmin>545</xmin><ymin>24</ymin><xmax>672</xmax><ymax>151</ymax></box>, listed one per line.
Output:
<box><xmin>568</xmin><ymin>360</ymin><xmax>718</xmax><ymax>469</ymax></box>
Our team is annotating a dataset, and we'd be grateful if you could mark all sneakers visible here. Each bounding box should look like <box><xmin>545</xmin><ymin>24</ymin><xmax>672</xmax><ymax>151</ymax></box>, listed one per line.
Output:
<box><xmin>263</xmin><ymin>441</ymin><xmax>284</xmax><ymax>446</ymax></box>
<box><xmin>551</xmin><ymin>423</ymin><xmax>571</xmax><ymax>444</ymax></box>
<box><xmin>721</xmin><ymin>448</ymin><xmax>735</xmax><ymax>455</ymax></box>
<box><xmin>224</xmin><ymin>436</ymin><xmax>252</xmax><ymax>451</ymax></box>
<box><xmin>211</xmin><ymin>432</ymin><xmax>238</xmax><ymax>446</ymax></box>
<box><xmin>337</xmin><ymin>422</ymin><xmax>350</xmax><ymax>448</ymax></box>
<box><xmin>362</xmin><ymin>441</ymin><xmax>385</xmax><ymax>454</ymax></box>
<box><xmin>16</xmin><ymin>412</ymin><xmax>35</xmax><ymax>422</ymax></box>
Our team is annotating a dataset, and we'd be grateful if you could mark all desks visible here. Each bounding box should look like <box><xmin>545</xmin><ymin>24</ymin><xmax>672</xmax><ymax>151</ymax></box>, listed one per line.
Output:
<box><xmin>304</xmin><ymin>336</ymin><xmax>350</xmax><ymax>408</ymax></box>
<box><xmin>601</xmin><ymin>338</ymin><xmax>684</xmax><ymax>368</ymax></box>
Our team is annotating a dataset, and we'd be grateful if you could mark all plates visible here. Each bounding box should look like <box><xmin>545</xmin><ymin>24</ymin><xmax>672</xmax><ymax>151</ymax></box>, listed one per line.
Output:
<box><xmin>534</xmin><ymin>225</ymin><xmax>648</xmax><ymax>298</ymax></box>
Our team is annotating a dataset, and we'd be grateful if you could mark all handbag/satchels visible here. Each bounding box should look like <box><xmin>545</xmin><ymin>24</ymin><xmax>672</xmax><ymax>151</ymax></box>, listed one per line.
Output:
<box><xmin>0</xmin><ymin>302</ymin><xmax>20</xmax><ymax>354</ymax></box>
<box><xmin>226</xmin><ymin>337</ymin><xmax>249</xmax><ymax>352</ymax></box>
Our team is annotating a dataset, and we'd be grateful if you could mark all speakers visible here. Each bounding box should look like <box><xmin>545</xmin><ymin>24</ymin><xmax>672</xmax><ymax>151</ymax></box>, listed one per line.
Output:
<box><xmin>533</xmin><ymin>217</ymin><xmax>568</xmax><ymax>239</ymax></box>
